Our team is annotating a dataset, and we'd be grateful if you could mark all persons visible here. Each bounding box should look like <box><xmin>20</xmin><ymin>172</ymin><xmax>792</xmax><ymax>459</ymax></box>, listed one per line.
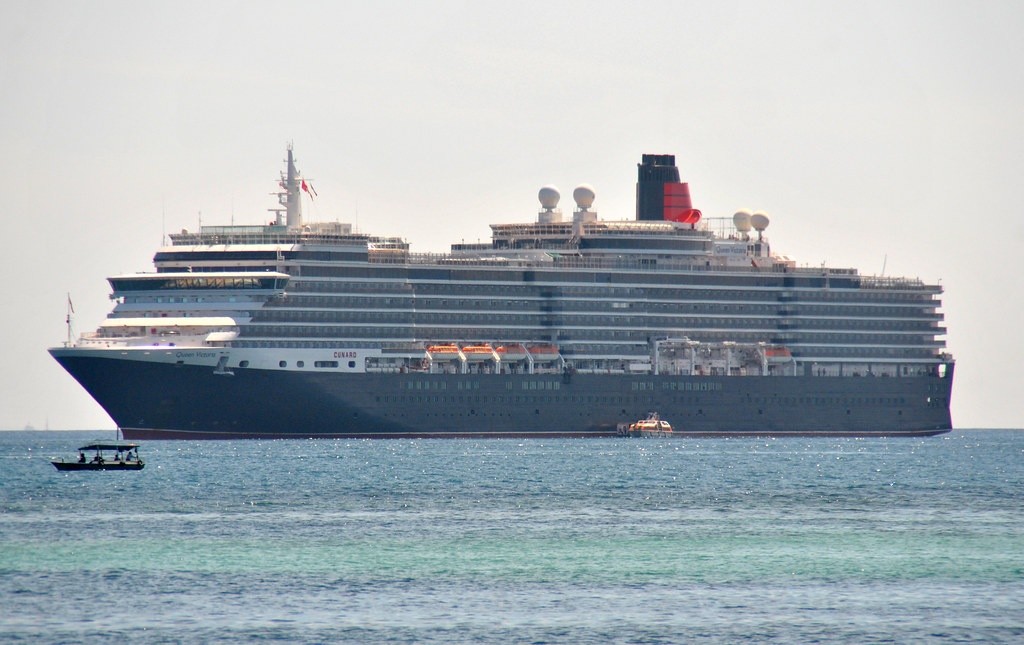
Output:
<box><xmin>126</xmin><ymin>451</ymin><xmax>137</xmax><ymax>461</ymax></box>
<box><xmin>94</xmin><ymin>453</ymin><xmax>103</xmax><ymax>460</ymax></box>
<box><xmin>79</xmin><ymin>453</ymin><xmax>86</xmax><ymax>462</ymax></box>
<box><xmin>114</xmin><ymin>454</ymin><xmax>121</xmax><ymax>461</ymax></box>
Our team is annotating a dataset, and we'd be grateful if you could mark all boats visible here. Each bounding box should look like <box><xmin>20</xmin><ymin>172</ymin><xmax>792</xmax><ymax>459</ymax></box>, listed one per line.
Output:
<box><xmin>495</xmin><ymin>343</ymin><xmax>527</xmax><ymax>363</ymax></box>
<box><xmin>627</xmin><ymin>412</ymin><xmax>673</xmax><ymax>439</ymax></box>
<box><xmin>428</xmin><ymin>344</ymin><xmax>459</xmax><ymax>360</ymax></box>
<box><xmin>461</xmin><ymin>342</ymin><xmax>494</xmax><ymax>363</ymax></box>
<box><xmin>528</xmin><ymin>344</ymin><xmax>559</xmax><ymax>363</ymax></box>
<box><xmin>47</xmin><ymin>440</ymin><xmax>146</xmax><ymax>471</ymax></box>
<box><xmin>767</xmin><ymin>347</ymin><xmax>792</xmax><ymax>367</ymax></box>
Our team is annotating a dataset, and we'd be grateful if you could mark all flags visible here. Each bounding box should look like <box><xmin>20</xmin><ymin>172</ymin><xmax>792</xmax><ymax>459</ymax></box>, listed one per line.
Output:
<box><xmin>302</xmin><ymin>179</ymin><xmax>309</xmax><ymax>191</ymax></box>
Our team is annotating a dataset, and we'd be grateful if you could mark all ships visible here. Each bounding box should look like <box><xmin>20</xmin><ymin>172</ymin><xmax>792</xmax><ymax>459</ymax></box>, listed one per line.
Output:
<box><xmin>45</xmin><ymin>134</ymin><xmax>956</xmax><ymax>441</ymax></box>
